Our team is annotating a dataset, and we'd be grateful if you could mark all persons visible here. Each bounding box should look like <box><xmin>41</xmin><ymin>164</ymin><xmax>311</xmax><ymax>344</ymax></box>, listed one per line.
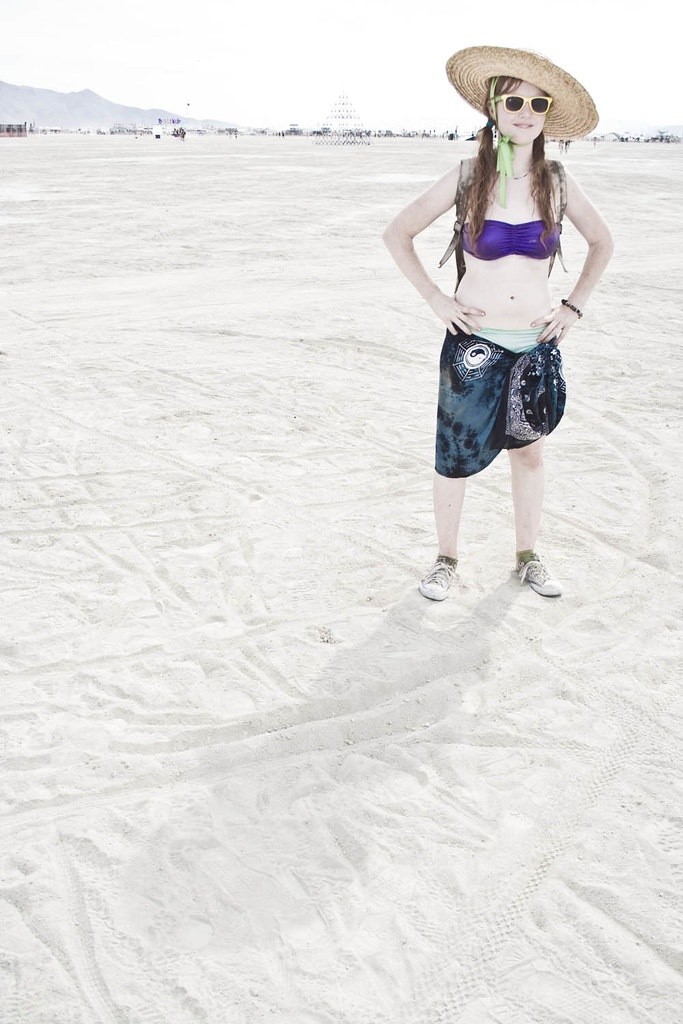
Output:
<box><xmin>383</xmin><ymin>45</ymin><xmax>616</xmax><ymax>601</ymax></box>
<box><xmin>7</xmin><ymin>117</ymin><xmax>669</xmax><ymax>149</ymax></box>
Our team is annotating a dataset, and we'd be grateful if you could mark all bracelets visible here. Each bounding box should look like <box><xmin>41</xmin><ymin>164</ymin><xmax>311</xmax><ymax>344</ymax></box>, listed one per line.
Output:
<box><xmin>562</xmin><ymin>298</ymin><xmax>583</xmax><ymax>319</ymax></box>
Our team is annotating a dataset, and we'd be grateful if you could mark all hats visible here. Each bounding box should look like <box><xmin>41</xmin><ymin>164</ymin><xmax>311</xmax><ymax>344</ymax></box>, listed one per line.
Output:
<box><xmin>445</xmin><ymin>43</ymin><xmax>598</xmax><ymax>143</ymax></box>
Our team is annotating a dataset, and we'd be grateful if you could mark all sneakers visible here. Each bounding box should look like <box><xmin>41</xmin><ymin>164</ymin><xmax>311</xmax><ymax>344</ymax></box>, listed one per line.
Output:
<box><xmin>516</xmin><ymin>555</ymin><xmax>562</xmax><ymax>599</ymax></box>
<box><xmin>418</xmin><ymin>559</ymin><xmax>460</xmax><ymax>602</ymax></box>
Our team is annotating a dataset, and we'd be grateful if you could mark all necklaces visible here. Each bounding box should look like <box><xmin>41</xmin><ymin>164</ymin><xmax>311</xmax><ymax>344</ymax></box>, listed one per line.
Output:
<box><xmin>505</xmin><ymin>172</ymin><xmax>529</xmax><ymax>179</ymax></box>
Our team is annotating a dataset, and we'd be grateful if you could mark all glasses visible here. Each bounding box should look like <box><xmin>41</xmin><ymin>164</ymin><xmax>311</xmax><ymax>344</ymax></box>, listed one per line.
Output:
<box><xmin>491</xmin><ymin>93</ymin><xmax>553</xmax><ymax>117</ymax></box>
